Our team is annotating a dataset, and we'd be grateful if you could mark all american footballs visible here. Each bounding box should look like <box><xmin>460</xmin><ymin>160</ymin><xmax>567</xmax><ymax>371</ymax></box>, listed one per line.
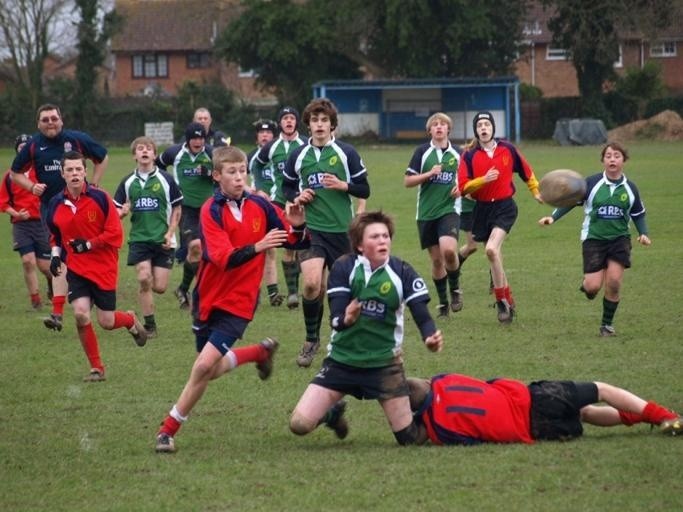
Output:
<box><xmin>539</xmin><ymin>169</ymin><xmax>587</xmax><ymax>209</ymax></box>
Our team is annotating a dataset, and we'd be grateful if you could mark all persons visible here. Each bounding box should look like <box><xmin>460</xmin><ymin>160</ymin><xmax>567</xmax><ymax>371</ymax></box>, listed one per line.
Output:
<box><xmin>459</xmin><ymin>194</ymin><xmax>479</xmax><ymax>264</ymax></box>
<box><xmin>252</xmin><ymin>107</ymin><xmax>309</xmax><ymax>307</ymax></box>
<box><xmin>405</xmin><ymin>113</ymin><xmax>464</xmax><ymax>318</ymax></box>
<box><xmin>156</xmin><ymin>147</ymin><xmax>289</xmax><ymax>452</ymax></box>
<box><xmin>10</xmin><ymin>104</ymin><xmax>110</xmax><ymax>331</ymax></box>
<box><xmin>0</xmin><ymin>135</ymin><xmax>55</xmax><ymax>308</ymax></box>
<box><xmin>114</xmin><ymin>135</ymin><xmax>184</xmax><ymax>340</ymax></box>
<box><xmin>288</xmin><ymin>212</ymin><xmax>443</xmax><ymax>444</ymax></box>
<box><xmin>319</xmin><ymin>198</ymin><xmax>367</xmax><ymax>321</ymax></box>
<box><xmin>406</xmin><ymin>377</ymin><xmax>683</xmax><ymax>445</ymax></box>
<box><xmin>539</xmin><ymin>141</ymin><xmax>651</xmax><ymax>338</ymax></box>
<box><xmin>46</xmin><ymin>153</ymin><xmax>146</xmax><ymax>381</ymax></box>
<box><xmin>247</xmin><ymin>119</ymin><xmax>283</xmax><ymax>197</ymax></box>
<box><xmin>456</xmin><ymin>112</ymin><xmax>543</xmax><ymax>322</ymax></box>
<box><xmin>194</xmin><ymin>107</ymin><xmax>232</xmax><ymax>148</ymax></box>
<box><xmin>282</xmin><ymin>98</ymin><xmax>371</xmax><ymax>366</ymax></box>
<box><xmin>155</xmin><ymin>123</ymin><xmax>218</xmax><ymax>309</ymax></box>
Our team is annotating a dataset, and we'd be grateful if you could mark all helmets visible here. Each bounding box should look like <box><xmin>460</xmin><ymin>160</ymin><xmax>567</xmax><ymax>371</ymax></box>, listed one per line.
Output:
<box><xmin>185</xmin><ymin>123</ymin><xmax>206</xmax><ymax>144</ymax></box>
<box><xmin>14</xmin><ymin>135</ymin><xmax>32</xmax><ymax>155</ymax></box>
<box><xmin>473</xmin><ymin>113</ymin><xmax>495</xmax><ymax>137</ymax></box>
<box><xmin>256</xmin><ymin>122</ymin><xmax>276</xmax><ymax>139</ymax></box>
<box><xmin>278</xmin><ymin>107</ymin><xmax>298</xmax><ymax>127</ymax></box>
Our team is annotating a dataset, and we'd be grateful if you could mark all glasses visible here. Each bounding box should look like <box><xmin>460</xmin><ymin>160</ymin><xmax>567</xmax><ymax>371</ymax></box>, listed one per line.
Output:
<box><xmin>39</xmin><ymin>117</ymin><xmax>60</xmax><ymax>122</ymax></box>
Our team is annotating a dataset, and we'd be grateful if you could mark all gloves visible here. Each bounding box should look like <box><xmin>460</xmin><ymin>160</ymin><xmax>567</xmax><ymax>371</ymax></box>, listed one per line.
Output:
<box><xmin>50</xmin><ymin>256</ymin><xmax>61</xmax><ymax>277</ymax></box>
<box><xmin>69</xmin><ymin>239</ymin><xmax>89</xmax><ymax>254</ymax></box>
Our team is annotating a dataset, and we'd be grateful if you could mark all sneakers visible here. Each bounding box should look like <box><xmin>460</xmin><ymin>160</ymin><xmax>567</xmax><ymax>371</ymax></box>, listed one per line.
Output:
<box><xmin>488</xmin><ymin>287</ymin><xmax>494</xmax><ymax>295</ymax></box>
<box><xmin>286</xmin><ymin>294</ymin><xmax>299</xmax><ymax>308</ymax></box>
<box><xmin>511</xmin><ymin>302</ymin><xmax>516</xmax><ymax>310</ymax></box>
<box><xmin>435</xmin><ymin>302</ymin><xmax>449</xmax><ymax>318</ymax></box>
<box><xmin>493</xmin><ymin>298</ymin><xmax>513</xmax><ymax>322</ymax></box>
<box><xmin>25</xmin><ymin>302</ymin><xmax>43</xmax><ymax>312</ymax></box>
<box><xmin>296</xmin><ymin>341</ymin><xmax>320</xmax><ymax>367</ymax></box>
<box><xmin>599</xmin><ymin>324</ymin><xmax>617</xmax><ymax>336</ymax></box>
<box><xmin>45</xmin><ymin>299</ymin><xmax>52</xmax><ymax>306</ymax></box>
<box><xmin>579</xmin><ymin>285</ymin><xmax>596</xmax><ymax>300</ymax></box>
<box><xmin>255</xmin><ymin>336</ymin><xmax>279</xmax><ymax>380</ymax></box>
<box><xmin>143</xmin><ymin>323</ymin><xmax>157</xmax><ymax>339</ymax></box>
<box><xmin>127</xmin><ymin>310</ymin><xmax>148</xmax><ymax>346</ymax></box>
<box><xmin>268</xmin><ymin>292</ymin><xmax>285</xmax><ymax>306</ymax></box>
<box><xmin>449</xmin><ymin>289</ymin><xmax>463</xmax><ymax>312</ymax></box>
<box><xmin>174</xmin><ymin>289</ymin><xmax>190</xmax><ymax>309</ymax></box>
<box><xmin>44</xmin><ymin>313</ymin><xmax>63</xmax><ymax>332</ymax></box>
<box><xmin>82</xmin><ymin>368</ymin><xmax>106</xmax><ymax>382</ymax></box>
<box><xmin>661</xmin><ymin>414</ymin><xmax>683</xmax><ymax>436</ymax></box>
<box><xmin>334</xmin><ymin>400</ymin><xmax>349</xmax><ymax>440</ymax></box>
<box><xmin>155</xmin><ymin>432</ymin><xmax>175</xmax><ymax>453</ymax></box>
